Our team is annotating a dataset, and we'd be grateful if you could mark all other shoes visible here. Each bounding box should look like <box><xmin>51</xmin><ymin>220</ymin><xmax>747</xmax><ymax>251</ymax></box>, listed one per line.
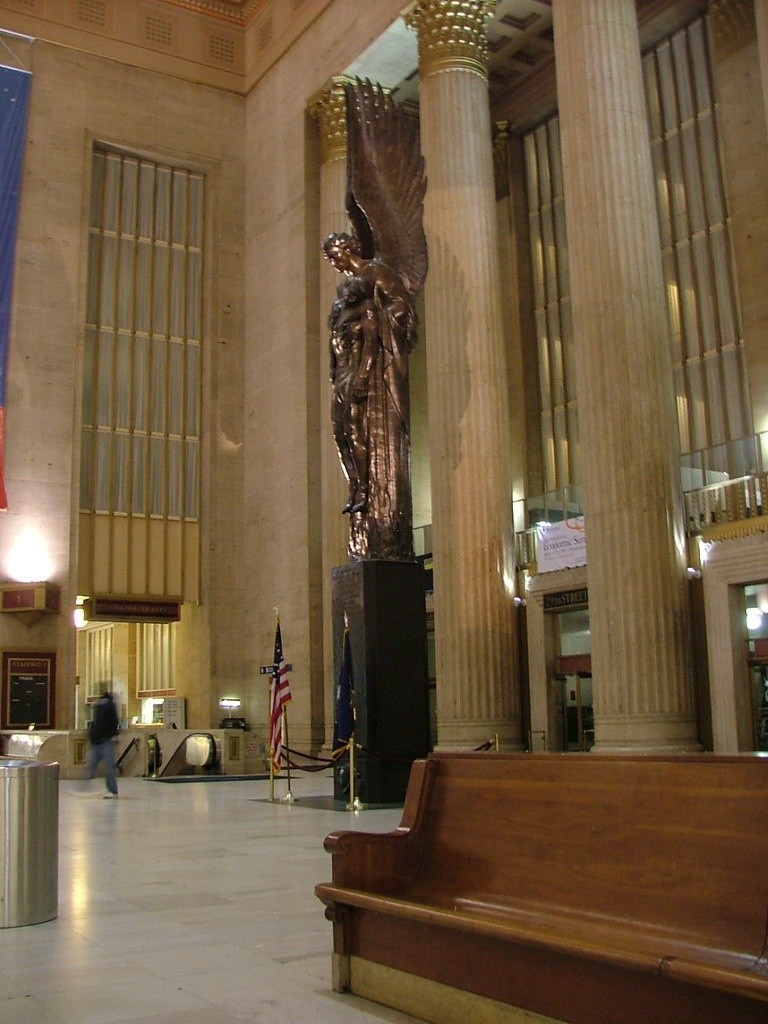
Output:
<box><xmin>103</xmin><ymin>791</ymin><xmax>118</xmax><ymax>799</ymax></box>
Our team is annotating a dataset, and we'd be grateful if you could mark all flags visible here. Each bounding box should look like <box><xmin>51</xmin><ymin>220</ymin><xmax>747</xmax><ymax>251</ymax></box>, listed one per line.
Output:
<box><xmin>331</xmin><ymin>624</ymin><xmax>358</xmax><ymax>761</ymax></box>
<box><xmin>269</xmin><ymin>617</ymin><xmax>292</xmax><ymax>773</ymax></box>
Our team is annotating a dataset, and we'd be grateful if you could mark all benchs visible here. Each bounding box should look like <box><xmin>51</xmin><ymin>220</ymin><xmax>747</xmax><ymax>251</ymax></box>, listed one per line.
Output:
<box><xmin>315</xmin><ymin>752</ymin><xmax>768</xmax><ymax>1024</ymax></box>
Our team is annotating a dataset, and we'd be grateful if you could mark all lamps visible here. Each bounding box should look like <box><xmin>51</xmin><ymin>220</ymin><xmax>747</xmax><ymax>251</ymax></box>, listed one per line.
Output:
<box><xmin>220</xmin><ymin>698</ymin><xmax>240</xmax><ymax>718</ymax></box>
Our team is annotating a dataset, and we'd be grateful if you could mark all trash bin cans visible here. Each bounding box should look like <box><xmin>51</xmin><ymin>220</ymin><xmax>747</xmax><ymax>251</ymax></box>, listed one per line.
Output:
<box><xmin>0</xmin><ymin>759</ymin><xmax>61</xmax><ymax>929</ymax></box>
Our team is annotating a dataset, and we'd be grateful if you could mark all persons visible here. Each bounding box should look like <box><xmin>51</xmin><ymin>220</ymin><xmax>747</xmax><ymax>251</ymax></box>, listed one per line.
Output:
<box><xmin>63</xmin><ymin>681</ymin><xmax>121</xmax><ymax>800</ymax></box>
<box><xmin>319</xmin><ymin>232</ymin><xmax>417</xmax><ymax>515</ymax></box>
